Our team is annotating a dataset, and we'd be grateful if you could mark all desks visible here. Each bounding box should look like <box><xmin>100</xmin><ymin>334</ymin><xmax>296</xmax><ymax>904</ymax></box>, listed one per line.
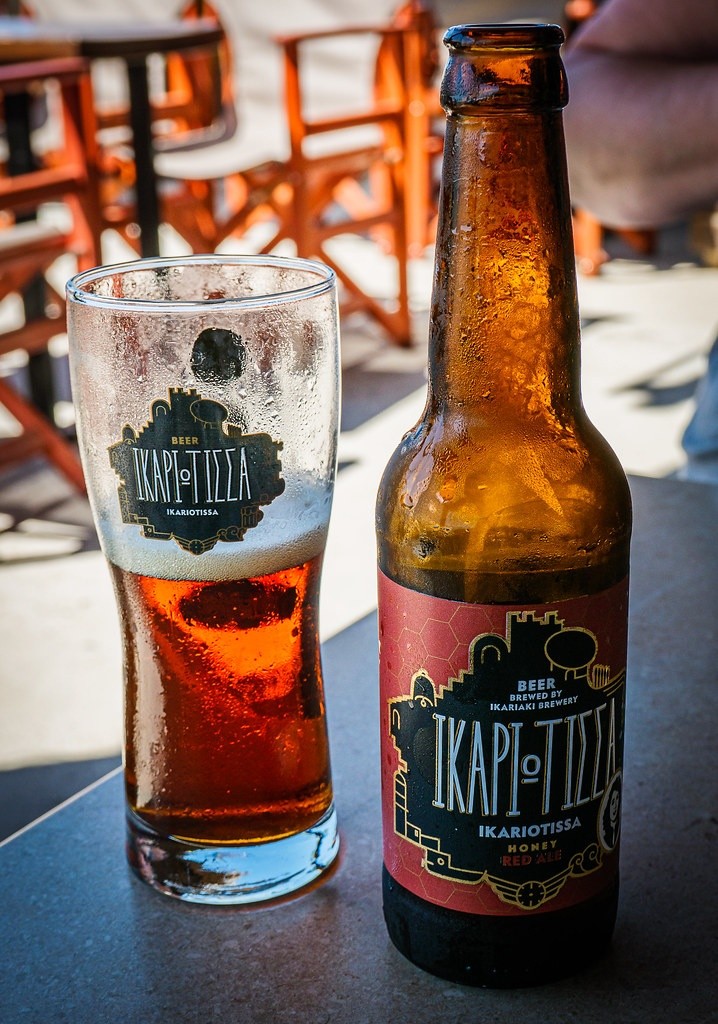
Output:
<box><xmin>2</xmin><ymin>17</ymin><xmax>223</xmax><ymax>259</ymax></box>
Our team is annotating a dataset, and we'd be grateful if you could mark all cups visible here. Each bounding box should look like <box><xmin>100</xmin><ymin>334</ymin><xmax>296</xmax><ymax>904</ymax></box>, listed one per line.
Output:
<box><xmin>66</xmin><ymin>254</ymin><xmax>342</xmax><ymax>906</ymax></box>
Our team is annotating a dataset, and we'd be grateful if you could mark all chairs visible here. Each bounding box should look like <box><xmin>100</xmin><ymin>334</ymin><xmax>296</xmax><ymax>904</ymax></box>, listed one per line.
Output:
<box><xmin>1</xmin><ymin>55</ymin><xmax>103</xmax><ymax>495</ymax></box>
<box><xmin>83</xmin><ymin>1</ymin><xmax>237</xmax><ymax>303</ymax></box>
<box><xmin>117</xmin><ymin>22</ymin><xmax>414</xmax><ymax>354</ymax></box>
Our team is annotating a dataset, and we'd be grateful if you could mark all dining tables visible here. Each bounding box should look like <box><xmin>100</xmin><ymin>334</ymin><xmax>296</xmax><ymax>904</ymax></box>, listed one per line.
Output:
<box><xmin>1</xmin><ymin>471</ymin><xmax>718</xmax><ymax>1024</ymax></box>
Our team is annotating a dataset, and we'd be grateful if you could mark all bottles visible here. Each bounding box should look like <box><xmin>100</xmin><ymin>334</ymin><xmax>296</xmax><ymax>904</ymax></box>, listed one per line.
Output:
<box><xmin>374</xmin><ymin>24</ymin><xmax>632</xmax><ymax>992</ymax></box>
